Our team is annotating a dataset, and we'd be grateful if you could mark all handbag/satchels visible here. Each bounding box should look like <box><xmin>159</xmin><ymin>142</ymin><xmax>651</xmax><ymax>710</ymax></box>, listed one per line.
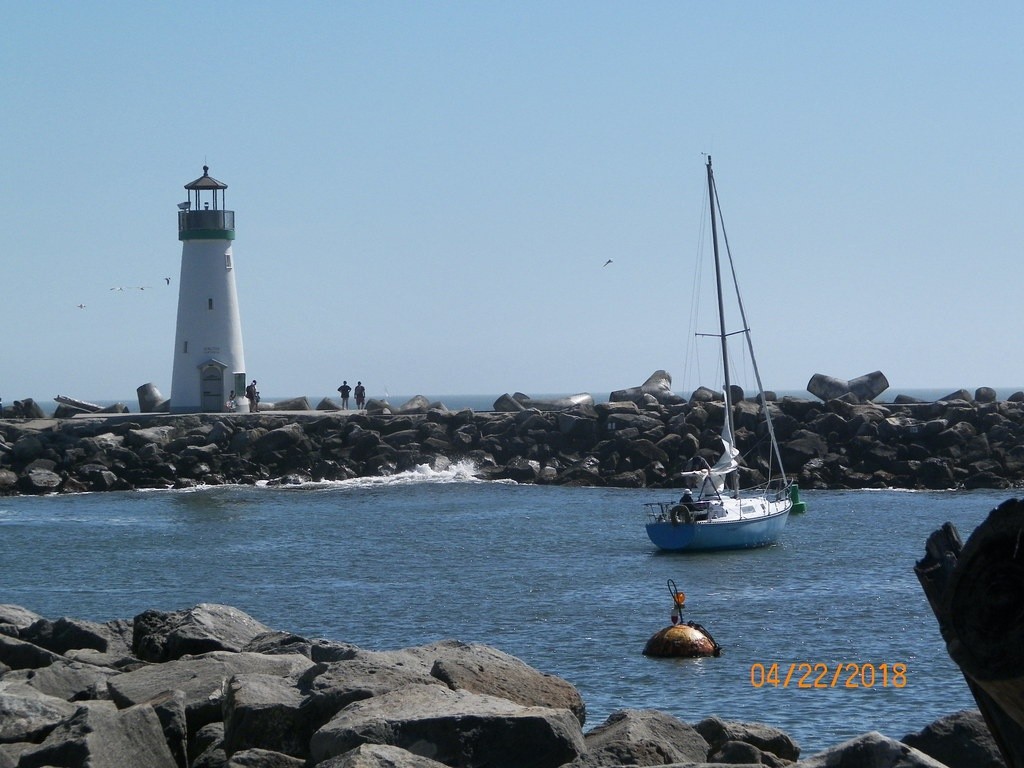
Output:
<box><xmin>257</xmin><ymin>393</ymin><xmax>261</xmax><ymax>402</ymax></box>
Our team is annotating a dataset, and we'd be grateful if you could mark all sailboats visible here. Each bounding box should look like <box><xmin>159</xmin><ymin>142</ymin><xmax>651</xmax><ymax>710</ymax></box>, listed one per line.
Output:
<box><xmin>644</xmin><ymin>152</ymin><xmax>803</xmax><ymax>555</ymax></box>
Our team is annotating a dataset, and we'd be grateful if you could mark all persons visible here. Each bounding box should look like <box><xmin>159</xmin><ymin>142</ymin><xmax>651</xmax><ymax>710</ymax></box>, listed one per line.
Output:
<box><xmin>228</xmin><ymin>391</ymin><xmax>237</xmax><ymax>413</ymax></box>
<box><xmin>354</xmin><ymin>382</ymin><xmax>365</xmax><ymax>410</ymax></box>
<box><xmin>338</xmin><ymin>381</ymin><xmax>351</xmax><ymax>410</ymax></box>
<box><xmin>677</xmin><ymin>489</ymin><xmax>706</xmax><ymax>520</ymax></box>
<box><xmin>245</xmin><ymin>380</ymin><xmax>261</xmax><ymax>413</ymax></box>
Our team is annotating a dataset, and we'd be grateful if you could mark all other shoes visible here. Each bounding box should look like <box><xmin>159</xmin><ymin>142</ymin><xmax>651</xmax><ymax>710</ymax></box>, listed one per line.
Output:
<box><xmin>250</xmin><ymin>411</ymin><xmax>255</xmax><ymax>413</ymax></box>
<box><xmin>256</xmin><ymin>409</ymin><xmax>260</xmax><ymax>412</ymax></box>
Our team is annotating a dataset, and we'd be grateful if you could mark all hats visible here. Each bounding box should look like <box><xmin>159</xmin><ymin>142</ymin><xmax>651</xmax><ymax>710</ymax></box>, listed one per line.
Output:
<box><xmin>683</xmin><ymin>488</ymin><xmax>692</xmax><ymax>495</ymax></box>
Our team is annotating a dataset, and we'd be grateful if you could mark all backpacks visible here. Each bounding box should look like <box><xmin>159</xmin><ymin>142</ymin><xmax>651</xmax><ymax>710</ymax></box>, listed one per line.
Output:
<box><xmin>245</xmin><ymin>384</ymin><xmax>256</xmax><ymax>399</ymax></box>
